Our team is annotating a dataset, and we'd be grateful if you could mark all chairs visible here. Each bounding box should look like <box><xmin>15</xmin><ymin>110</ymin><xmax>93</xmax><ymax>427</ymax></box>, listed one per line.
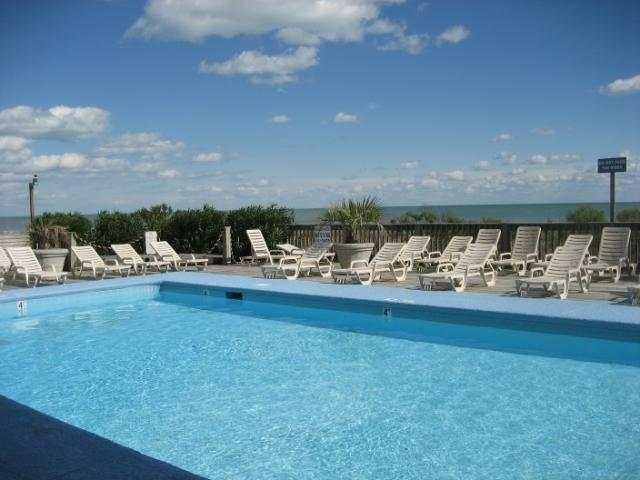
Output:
<box><xmin>276</xmin><ymin>244</ymin><xmax>336</xmax><ymax>264</ymax></box>
<box><xmin>111</xmin><ymin>244</ymin><xmax>171</xmax><ymax>275</ymax></box>
<box><xmin>331</xmin><ymin>243</ymin><xmax>407</xmax><ymax>285</ymax></box>
<box><xmin>246</xmin><ymin>230</ymin><xmax>301</xmax><ymax>272</ymax></box>
<box><xmin>1</xmin><ymin>249</ymin><xmax>14</xmax><ymax>278</ymax></box>
<box><xmin>71</xmin><ymin>246</ymin><xmax>131</xmax><ymax>280</ymax></box>
<box><xmin>397</xmin><ymin>226</ymin><xmax>593</xmax><ymax>277</ymax></box>
<box><xmin>589</xmin><ymin>227</ymin><xmax>635</xmax><ymax>283</ymax></box>
<box><xmin>418</xmin><ymin>244</ymin><xmax>497</xmax><ymax>292</ymax></box>
<box><xmin>516</xmin><ymin>245</ymin><xmax>591</xmax><ymax>298</ymax></box>
<box><xmin>6</xmin><ymin>247</ymin><xmax>68</xmax><ymax>288</ymax></box>
<box><xmin>261</xmin><ymin>241</ymin><xmax>333</xmax><ymax>280</ymax></box>
<box><xmin>145</xmin><ymin>231</ymin><xmax>160</xmax><ymax>261</ymax></box>
<box><xmin>149</xmin><ymin>241</ymin><xmax>208</xmax><ymax>272</ymax></box>
